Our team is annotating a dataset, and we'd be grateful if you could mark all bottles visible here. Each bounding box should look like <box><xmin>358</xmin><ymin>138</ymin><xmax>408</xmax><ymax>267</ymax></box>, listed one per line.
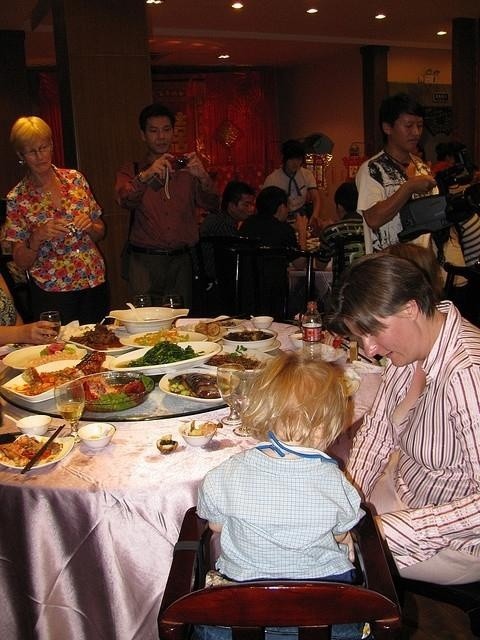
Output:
<box><xmin>302</xmin><ymin>301</ymin><xmax>322</xmax><ymax>361</ymax></box>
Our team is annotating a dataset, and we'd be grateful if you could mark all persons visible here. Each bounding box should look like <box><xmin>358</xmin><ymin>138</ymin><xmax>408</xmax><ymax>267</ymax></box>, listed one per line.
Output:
<box><xmin>356</xmin><ymin>95</ymin><xmax>441</xmax><ymax>267</ymax></box>
<box><xmin>412</xmin><ymin>144</ymin><xmax>431</xmax><ymax>170</ymax></box>
<box><xmin>432</xmin><ymin>143</ymin><xmax>454</xmax><ymax>173</ymax></box>
<box><xmin>238</xmin><ymin>186</ymin><xmax>308</xmax><ymax>321</ymax></box>
<box><xmin>324</xmin><ymin>253</ymin><xmax>480</xmax><ymax>584</ymax></box>
<box><xmin>113</xmin><ymin>104</ymin><xmax>220</xmax><ymax>316</ymax></box>
<box><xmin>3</xmin><ymin>116</ymin><xmax>110</xmax><ymax>326</ymax></box>
<box><xmin>262</xmin><ymin>138</ymin><xmax>322</xmax><ymax>227</ymax></box>
<box><xmin>193</xmin><ymin>181</ymin><xmax>255</xmax><ymax>282</ymax></box>
<box><xmin>431</xmin><ymin>213</ymin><xmax>480</xmax><ymax>286</ymax></box>
<box><xmin>196</xmin><ymin>351</ymin><xmax>366</xmax><ymax>585</ymax></box>
<box><xmin>0</xmin><ymin>274</ymin><xmax>57</xmax><ymax>344</ymax></box>
<box><xmin>312</xmin><ymin>183</ymin><xmax>367</xmax><ymax>304</ymax></box>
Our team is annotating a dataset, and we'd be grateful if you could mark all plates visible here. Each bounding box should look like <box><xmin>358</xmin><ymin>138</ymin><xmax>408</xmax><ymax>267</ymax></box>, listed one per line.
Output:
<box><xmin>322</xmin><ymin>344</ymin><xmax>346</xmax><ymax>362</ymax></box>
<box><xmin>120</xmin><ymin>331</ymin><xmax>209</xmax><ymax>348</ymax></box>
<box><xmin>221</xmin><ymin>340</ymin><xmax>282</xmax><ymax>352</ymax></box>
<box><xmin>2</xmin><ymin>345</ymin><xmax>88</xmax><ymax>371</ymax></box>
<box><xmin>1</xmin><ymin>434</ymin><xmax>75</xmax><ymax>470</ymax></box>
<box><xmin>221</xmin><ymin>329</ymin><xmax>278</xmax><ymax>347</ymax></box>
<box><xmin>66</xmin><ymin>330</ymin><xmax>134</xmax><ymax>353</ymax></box>
<box><xmin>203</xmin><ymin>345</ymin><xmax>278</xmax><ymax>374</ymax></box>
<box><xmin>159</xmin><ymin>368</ymin><xmax>228</xmax><ymax>403</ymax></box>
<box><xmin>72</xmin><ymin>371</ymin><xmax>155</xmax><ymax>411</ymax></box>
<box><xmin>1</xmin><ymin>360</ymin><xmax>87</xmax><ymax>403</ymax></box>
<box><xmin>109</xmin><ymin>342</ymin><xmax>221</xmax><ymax>375</ymax></box>
<box><xmin>213</xmin><ymin>315</ymin><xmax>244</xmax><ymax>329</ymax></box>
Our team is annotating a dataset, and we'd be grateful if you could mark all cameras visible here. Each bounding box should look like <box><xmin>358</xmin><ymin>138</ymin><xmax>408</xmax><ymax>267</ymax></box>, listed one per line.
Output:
<box><xmin>167</xmin><ymin>154</ymin><xmax>188</xmax><ymax>170</ymax></box>
<box><xmin>397</xmin><ymin>163</ymin><xmax>480</xmax><ymax>244</ymax></box>
<box><xmin>63</xmin><ymin>223</ymin><xmax>78</xmax><ymax>234</ymax></box>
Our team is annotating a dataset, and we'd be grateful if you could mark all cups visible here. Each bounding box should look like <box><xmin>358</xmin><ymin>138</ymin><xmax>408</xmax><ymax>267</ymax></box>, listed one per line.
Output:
<box><xmin>135</xmin><ymin>294</ymin><xmax>151</xmax><ymax>308</ymax></box>
<box><xmin>218</xmin><ymin>363</ymin><xmax>248</xmax><ymax>425</ymax></box>
<box><xmin>40</xmin><ymin>311</ymin><xmax>61</xmax><ymax>342</ymax></box>
<box><xmin>54</xmin><ymin>380</ymin><xmax>85</xmax><ymax>443</ymax></box>
<box><xmin>229</xmin><ymin>374</ymin><xmax>258</xmax><ymax>437</ymax></box>
<box><xmin>163</xmin><ymin>294</ymin><xmax>185</xmax><ymax>324</ymax></box>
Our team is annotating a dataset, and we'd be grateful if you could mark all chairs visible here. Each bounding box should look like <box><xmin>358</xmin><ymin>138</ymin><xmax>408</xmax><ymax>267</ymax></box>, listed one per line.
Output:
<box><xmin>443</xmin><ymin>262</ymin><xmax>479</xmax><ymax>327</ymax></box>
<box><xmin>199</xmin><ymin>235</ymin><xmax>255</xmax><ymax>311</ymax></box>
<box><xmin>158</xmin><ymin>504</ymin><xmax>402</xmax><ymax>640</ymax></box>
<box><xmin>402</xmin><ymin>576</ymin><xmax>480</xmax><ymax>640</ymax></box>
<box><xmin>228</xmin><ymin>244</ymin><xmax>320</xmax><ymax>324</ymax></box>
<box><xmin>0</xmin><ymin>255</ymin><xmax>36</xmax><ymax>325</ymax></box>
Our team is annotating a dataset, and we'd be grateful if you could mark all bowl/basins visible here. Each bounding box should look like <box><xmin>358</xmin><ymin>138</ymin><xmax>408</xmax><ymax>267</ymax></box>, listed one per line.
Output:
<box><xmin>110</xmin><ymin>307</ymin><xmax>189</xmax><ymax>334</ymax></box>
<box><xmin>16</xmin><ymin>415</ymin><xmax>51</xmax><ymax>435</ymax></box>
<box><xmin>78</xmin><ymin>423</ymin><xmax>116</xmax><ymax>449</ymax></box>
<box><xmin>181</xmin><ymin>420</ymin><xmax>217</xmax><ymax>447</ymax></box>
<box><xmin>290</xmin><ymin>334</ymin><xmax>304</xmax><ymax>348</ymax></box>
<box><xmin>251</xmin><ymin>316</ymin><xmax>273</xmax><ymax>328</ymax></box>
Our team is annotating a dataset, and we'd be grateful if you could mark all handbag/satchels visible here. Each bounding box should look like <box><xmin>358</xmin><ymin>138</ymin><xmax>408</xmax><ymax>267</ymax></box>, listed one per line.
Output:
<box><xmin>119</xmin><ymin>162</ymin><xmax>139</xmax><ymax>281</ymax></box>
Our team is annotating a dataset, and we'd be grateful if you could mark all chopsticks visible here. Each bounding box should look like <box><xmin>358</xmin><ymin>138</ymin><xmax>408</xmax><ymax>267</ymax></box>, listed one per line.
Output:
<box><xmin>21</xmin><ymin>424</ymin><xmax>70</xmax><ymax>475</ymax></box>
<box><xmin>343</xmin><ymin>343</ymin><xmax>373</xmax><ymax>364</ymax></box>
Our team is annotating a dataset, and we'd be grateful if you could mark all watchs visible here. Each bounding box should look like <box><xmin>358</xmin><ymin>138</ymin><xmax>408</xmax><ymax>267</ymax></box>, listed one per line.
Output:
<box><xmin>137</xmin><ymin>172</ymin><xmax>144</xmax><ymax>184</ymax></box>
<box><xmin>25</xmin><ymin>239</ymin><xmax>38</xmax><ymax>253</ymax></box>
